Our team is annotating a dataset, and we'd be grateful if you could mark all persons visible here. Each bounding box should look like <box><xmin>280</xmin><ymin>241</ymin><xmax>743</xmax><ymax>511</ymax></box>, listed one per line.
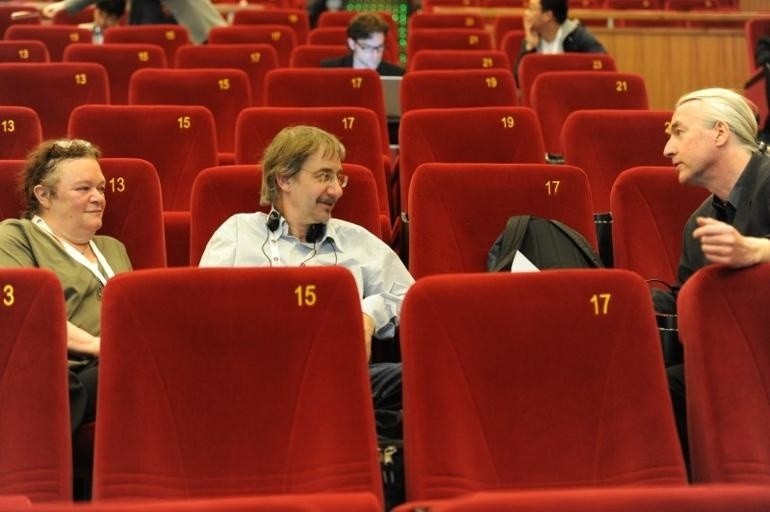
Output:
<box><xmin>45</xmin><ymin>1</ymin><xmax>225</xmax><ymax>43</ymax></box>
<box><xmin>79</xmin><ymin>1</ymin><xmax>126</xmax><ymax>36</ymax></box>
<box><xmin>524</xmin><ymin>0</ymin><xmax>605</xmax><ymax>57</ymax></box>
<box><xmin>322</xmin><ymin>13</ymin><xmax>406</xmax><ymax>78</ymax></box>
<box><xmin>198</xmin><ymin>124</ymin><xmax>414</xmax><ymax>439</ymax></box>
<box><xmin>0</xmin><ymin>140</ymin><xmax>135</xmax><ymax>440</ymax></box>
<box><xmin>664</xmin><ymin>87</ymin><xmax>770</xmax><ymax>284</ymax></box>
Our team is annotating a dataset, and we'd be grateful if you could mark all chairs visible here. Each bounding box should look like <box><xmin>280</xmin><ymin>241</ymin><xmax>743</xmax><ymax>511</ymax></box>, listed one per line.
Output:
<box><xmin>0</xmin><ymin>0</ymin><xmax>770</xmax><ymax>512</ymax></box>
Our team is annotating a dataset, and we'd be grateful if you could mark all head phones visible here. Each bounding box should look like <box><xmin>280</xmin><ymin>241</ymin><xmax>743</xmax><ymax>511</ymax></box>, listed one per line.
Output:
<box><xmin>266</xmin><ymin>211</ymin><xmax>325</xmax><ymax>244</ymax></box>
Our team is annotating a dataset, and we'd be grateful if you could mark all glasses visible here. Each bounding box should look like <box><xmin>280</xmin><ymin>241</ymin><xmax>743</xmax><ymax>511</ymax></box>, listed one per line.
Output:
<box><xmin>49</xmin><ymin>139</ymin><xmax>91</xmax><ymax>157</ymax></box>
<box><xmin>301</xmin><ymin>167</ymin><xmax>349</xmax><ymax>188</ymax></box>
<box><xmin>355</xmin><ymin>39</ymin><xmax>386</xmax><ymax>52</ymax></box>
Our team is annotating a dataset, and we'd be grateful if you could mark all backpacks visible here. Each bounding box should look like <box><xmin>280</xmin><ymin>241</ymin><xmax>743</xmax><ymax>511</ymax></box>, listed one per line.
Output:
<box><xmin>488</xmin><ymin>214</ymin><xmax>605</xmax><ymax>273</ymax></box>
